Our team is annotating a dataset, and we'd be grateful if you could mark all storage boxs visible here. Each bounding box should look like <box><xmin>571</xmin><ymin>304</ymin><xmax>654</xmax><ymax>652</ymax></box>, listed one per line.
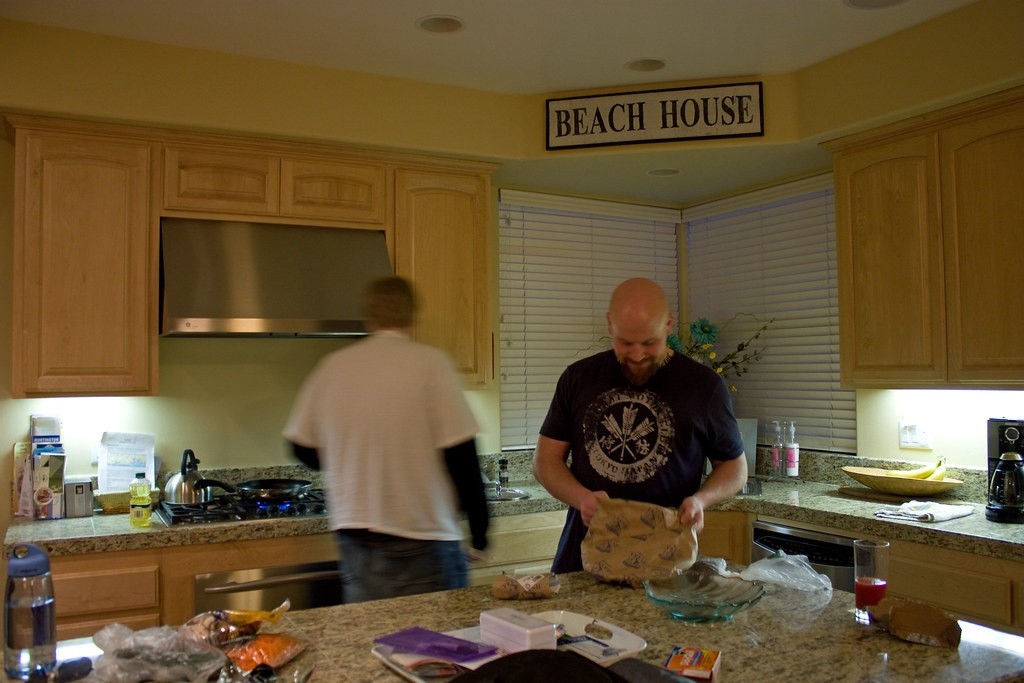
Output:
<box><xmin>664</xmin><ymin>646</ymin><xmax>722</xmax><ymax>683</ymax></box>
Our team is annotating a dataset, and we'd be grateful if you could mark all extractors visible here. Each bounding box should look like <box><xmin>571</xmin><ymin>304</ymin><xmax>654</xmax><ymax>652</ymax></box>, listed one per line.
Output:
<box><xmin>160</xmin><ymin>218</ymin><xmax>392</xmax><ymax>341</ymax></box>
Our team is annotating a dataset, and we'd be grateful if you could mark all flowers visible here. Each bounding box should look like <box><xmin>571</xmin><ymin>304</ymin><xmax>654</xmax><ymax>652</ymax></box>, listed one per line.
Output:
<box><xmin>666</xmin><ymin>310</ymin><xmax>779</xmax><ymax>393</ymax></box>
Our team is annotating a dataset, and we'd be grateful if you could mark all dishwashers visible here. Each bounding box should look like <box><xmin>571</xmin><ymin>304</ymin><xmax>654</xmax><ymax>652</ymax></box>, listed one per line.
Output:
<box><xmin>750</xmin><ymin>518</ymin><xmax>873</xmax><ymax>596</ymax></box>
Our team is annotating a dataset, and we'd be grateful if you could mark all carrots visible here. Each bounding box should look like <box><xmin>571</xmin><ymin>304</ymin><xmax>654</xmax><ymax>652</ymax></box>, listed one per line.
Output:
<box><xmin>226</xmin><ymin>633</ymin><xmax>305</xmax><ymax>671</ymax></box>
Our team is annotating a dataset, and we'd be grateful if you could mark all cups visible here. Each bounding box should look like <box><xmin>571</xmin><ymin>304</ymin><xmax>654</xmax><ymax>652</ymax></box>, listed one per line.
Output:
<box><xmin>853</xmin><ymin>538</ymin><xmax>890</xmax><ymax>626</ymax></box>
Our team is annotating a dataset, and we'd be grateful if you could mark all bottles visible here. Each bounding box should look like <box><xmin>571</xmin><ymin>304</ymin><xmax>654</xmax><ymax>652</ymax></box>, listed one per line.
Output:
<box><xmin>499</xmin><ymin>459</ymin><xmax>509</xmax><ymax>489</ymax></box>
<box><xmin>129</xmin><ymin>472</ymin><xmax>153</xmax><ymax>527</ymax></box>
<box><xmin>2</xmin><ymin>543</ymin><xmax>55</xmax><ymax>681</ymax></box>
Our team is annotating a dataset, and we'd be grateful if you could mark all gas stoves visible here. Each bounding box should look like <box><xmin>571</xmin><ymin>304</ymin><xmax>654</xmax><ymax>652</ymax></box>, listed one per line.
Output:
<box><xmin>154</xmin><ymin>489</ymin><xmax>327</xmax><ymax>529</ymax></box>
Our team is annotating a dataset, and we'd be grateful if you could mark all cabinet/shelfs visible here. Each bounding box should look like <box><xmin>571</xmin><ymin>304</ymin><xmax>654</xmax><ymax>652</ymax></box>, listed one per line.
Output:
<box><xmin>8</xmin><ymin>511</ymin><xmax>566</xmax><ymax>648</ymax></box>
<box><xmin>0</xmin><ymin>106</ymin><xmax>504</xmax><ymax>399</ymax></box>
<box><xmin>815</xmin><ymin>83</ymin><xmax>1024</xmax><ymax>392</ymax></box>
<box><xmin>698</xmin><ymin>514</ymin><xmax>1024</xmax><ymax>640</ymax></box>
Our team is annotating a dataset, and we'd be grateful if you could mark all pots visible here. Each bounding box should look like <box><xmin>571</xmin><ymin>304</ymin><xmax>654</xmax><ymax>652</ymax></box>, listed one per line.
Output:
<box><xmin>194</xmin><ymin>475</ymin><xmax>313</xmax><ymax>501</ymax></box>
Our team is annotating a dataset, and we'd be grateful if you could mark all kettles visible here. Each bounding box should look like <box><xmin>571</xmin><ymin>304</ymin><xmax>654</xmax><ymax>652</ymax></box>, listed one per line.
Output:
<box><xmin>164</xmin><ymin>449</ymin><xmax>213</xmax><ymax>505</ymax></box>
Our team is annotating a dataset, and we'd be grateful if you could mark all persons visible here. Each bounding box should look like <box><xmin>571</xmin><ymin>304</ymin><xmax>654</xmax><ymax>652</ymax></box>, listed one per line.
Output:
<box><xmin>281</xmin><ymin>277</ymin><xmax>490</xmax><ymax>604</ymax></box>
<box><xmin>532</xmin><ymin>277</ymin><xmax>748</xmax><ymax>575</ymax></box>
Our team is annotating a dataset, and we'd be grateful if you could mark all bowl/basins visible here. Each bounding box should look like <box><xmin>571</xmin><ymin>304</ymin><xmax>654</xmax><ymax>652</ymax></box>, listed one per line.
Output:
<box><xmin>641</xmin><ymin>573</ymin><xmax>766</xmax><ymax>626</ymax></box>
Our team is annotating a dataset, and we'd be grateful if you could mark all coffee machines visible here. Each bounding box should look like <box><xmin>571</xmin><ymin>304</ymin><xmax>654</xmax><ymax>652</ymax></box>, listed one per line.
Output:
<box><xmin>984</xmin><ymin>417</ymin><xmax>1024</xmax><ymax>525</ymax></box>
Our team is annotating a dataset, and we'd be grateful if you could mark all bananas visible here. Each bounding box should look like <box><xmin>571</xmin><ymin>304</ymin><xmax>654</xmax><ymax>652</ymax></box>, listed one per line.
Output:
<box><xmin>886</xmin><ymin>460</ymin><xmax>946</xmax><ymax>481</ymax></box>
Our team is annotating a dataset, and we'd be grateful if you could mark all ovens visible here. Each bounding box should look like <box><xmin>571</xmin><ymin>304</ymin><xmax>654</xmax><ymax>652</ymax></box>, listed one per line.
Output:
<box><xmin>194</xmin><ymin>562</ymin><xmax>343</xmax><ymax>616</ymax></box>
